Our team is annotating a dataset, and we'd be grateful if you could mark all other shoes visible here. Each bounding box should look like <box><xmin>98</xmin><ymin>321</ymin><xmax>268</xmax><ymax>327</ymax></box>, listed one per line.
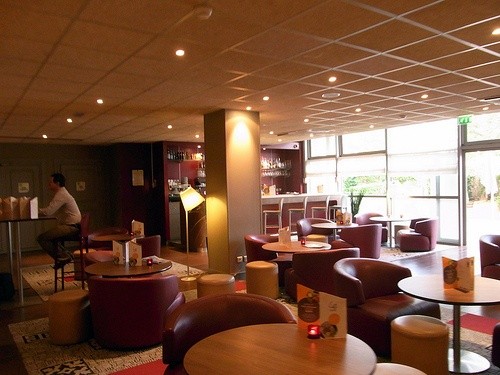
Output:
<box><xmin>53</xmin><ymin>252</ymin><xmax>74</xmax><ymax>269</ymax></box>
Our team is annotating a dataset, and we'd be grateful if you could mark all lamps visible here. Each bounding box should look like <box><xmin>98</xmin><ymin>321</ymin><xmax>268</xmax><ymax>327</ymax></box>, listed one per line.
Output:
<box><xmin>177</xmin><ymin>187</ymin><xmax>207</xmax><ymax>282</ymax></box>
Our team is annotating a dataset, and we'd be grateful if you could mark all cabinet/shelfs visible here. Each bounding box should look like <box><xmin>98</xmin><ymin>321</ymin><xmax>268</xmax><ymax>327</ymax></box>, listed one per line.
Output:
<box><xmin>258</xmin><ymin>144</ymin><xmax>304</xmax><ymax>194</ymax></box>
<box><xmin>169</xmin><ymin>143</ymin><xmax>206</xmax><ymax>194</ymax></box>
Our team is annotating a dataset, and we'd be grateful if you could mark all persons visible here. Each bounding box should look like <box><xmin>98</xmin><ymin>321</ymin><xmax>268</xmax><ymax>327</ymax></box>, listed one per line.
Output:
<box><xmin>38</xmin><ymin>172</ymin><xmax>82</xmax><ymax>269</ymax></box>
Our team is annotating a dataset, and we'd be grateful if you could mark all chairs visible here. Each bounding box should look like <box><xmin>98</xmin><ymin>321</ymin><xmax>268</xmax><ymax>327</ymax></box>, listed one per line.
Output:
<box><xmin>87</xmin><ymin>212</ymin><xmax>500</xmax><ymax>374</ymax></box>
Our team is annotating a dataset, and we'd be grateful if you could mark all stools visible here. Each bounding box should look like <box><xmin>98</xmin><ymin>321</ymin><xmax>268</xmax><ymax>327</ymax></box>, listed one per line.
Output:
<box><xmin>246</xmin><ymin>260</ymin><xmax>280</xmax><ymax>300</ymax></box>
<box><xmin>390</xmin><ymin>315</ymin><xmax>450</xmax><ymax>375</ymax></box>
<box><xmin>54</xmin><ymin>213</ymin><xmax>89</xmax><ymax>293</ymax></box>
<box><xmin>73</xmin><ymin>249</ymin><xmax>96</xmax><ymax>281</ymax></box>
<box><xmin>307</xmin><ymin>234</ymin><xmax>328</xmax><ymax>243</ymax></box>
<box><xmin>263</xmin><ymin>194</ymin><xmax>346</xmax><ymax>235</ymax></box>
<box><xmin>196</xmin><ymin>273</ymin><xmax>236</xmax><ymax>299</ymax></box>
<box><xmin>48</xmin><ymin>288</ymin><xmax>91</xmax><ymax>346</ymax></box>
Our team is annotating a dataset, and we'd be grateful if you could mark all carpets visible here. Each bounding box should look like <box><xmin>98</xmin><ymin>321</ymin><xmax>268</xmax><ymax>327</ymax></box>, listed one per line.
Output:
<box><xmin>8</xmin><ymin>280</ymin><xmax>499</xmax><ymax>375</ymax></box>
<box><xmin>17</xmin><ymin>255</ymin><xmax>205</xmax><ymax>301</ymax></box>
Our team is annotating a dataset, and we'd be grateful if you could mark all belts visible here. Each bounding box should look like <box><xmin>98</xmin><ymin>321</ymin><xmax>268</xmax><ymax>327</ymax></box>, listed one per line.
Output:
<box><xmin>64</xmin><ymin>223</ymin><xmax>76</xmax><ymax>227</ymax></box>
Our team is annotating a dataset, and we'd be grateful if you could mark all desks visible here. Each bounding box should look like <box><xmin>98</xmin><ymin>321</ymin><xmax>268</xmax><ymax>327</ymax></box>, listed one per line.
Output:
<box><xmin>311</xmin><ymin>222</ymin><xmax>358</xmax><ymax>241</ymax></box>
<box><xmin>183</xmin><ymin>323</ymin><xmax>376</xmax><ymax>375</ymax></box>
<box><xmin>84</xmin><ymin>258</ymin><xmax>172</xmax><ymax>278</ymax></box>
<box><xmin>397</xmin><ymin>274</ymin><xmax>500</xmax><ymax>373</ymax></box>
<box><xmin>0</xmin><ymin>217</ymin><xmax>56</xmax><ymax>303</ymax></box>
<box><xmin>369</xmin><ymin>217</ymin><xmax>410</xmax><ymax>249</ymax></box>
<box><xmin>89</xmin><ymin>234</ymin><xmax>131</xmax><ymax>245</ymax></box>
<box><xmin>262</xmin><ymin>239</ymin><xmax>332</xmax><ymax>267</ymax></box>
